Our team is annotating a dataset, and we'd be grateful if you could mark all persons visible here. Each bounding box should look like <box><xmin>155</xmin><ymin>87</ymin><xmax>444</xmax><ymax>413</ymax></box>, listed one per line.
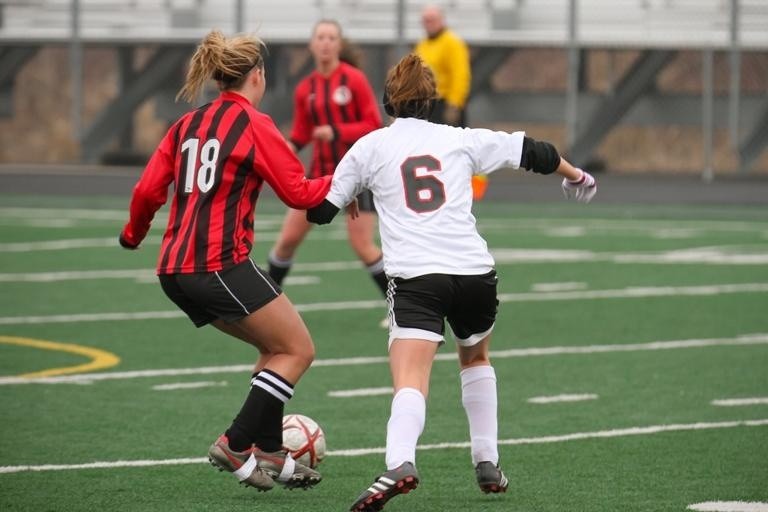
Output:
<box><xmin>268</xmin><ymin>20</ymin><xmax>389</xmax><ymax>331</ymax></box>
<box><xmin>413</xmin><ymin>6</ymin><xmax>472</xmax><ymax>129</ymax></box>
<box><xmin>119</xmin><ymin>27</ymin><xmax>359</xmax><ymax>492</ymax></box>
<box><xmin>306</xmin><ymin>56</ymin><xmax>597</xmax><ymax>512</ymax></box>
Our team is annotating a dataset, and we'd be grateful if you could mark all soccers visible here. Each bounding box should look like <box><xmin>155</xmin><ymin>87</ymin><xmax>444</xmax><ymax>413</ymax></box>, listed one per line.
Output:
<box><xmin>282</xmin><ymin>414</ymin><xmax>326</xmax><ymax>470</ymax></box>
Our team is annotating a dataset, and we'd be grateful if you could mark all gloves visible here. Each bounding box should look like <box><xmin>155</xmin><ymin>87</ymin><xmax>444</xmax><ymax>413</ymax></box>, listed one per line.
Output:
<box><xmin>561</xmin><ymin>167</ymin><xmax>597</xmax><ymax>203</ymax></box>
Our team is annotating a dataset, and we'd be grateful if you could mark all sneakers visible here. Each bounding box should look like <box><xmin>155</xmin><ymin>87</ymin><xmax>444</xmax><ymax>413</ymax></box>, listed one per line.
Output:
<box><xmin>474</xmin><ymin>460</ymin><xmax>508</xmax><ymax>494</ymax></box>
<box><xmin>207</xmin><ymin>432</ymin><xmax>275</xmax><ymax>493</ymax></box>
<box><xmin>252</xmin><ymin>444</ymin><xmax>322</xmax><ymax>491</ymax></box>
<box><xmin>349</xmin><ymin>461</ymin><xmax>420</xmax><ymax>512</ymax></box>
